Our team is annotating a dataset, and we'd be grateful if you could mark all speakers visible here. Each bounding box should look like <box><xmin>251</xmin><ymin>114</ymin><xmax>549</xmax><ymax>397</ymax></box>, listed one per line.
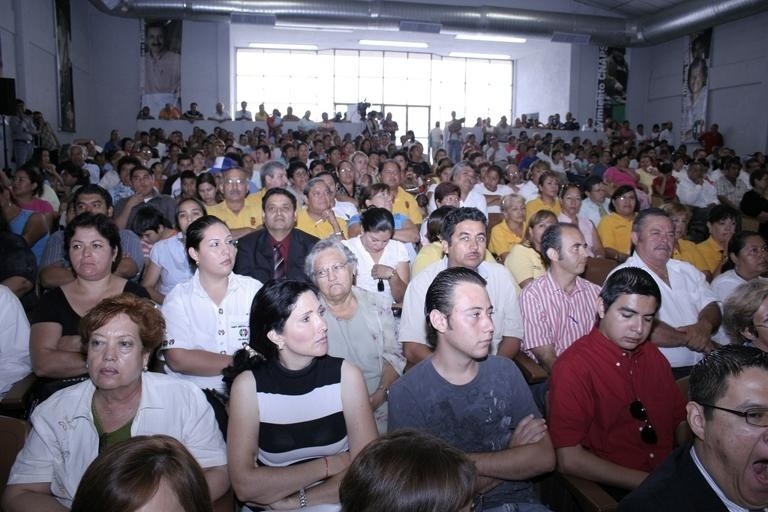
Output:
<box><xmin>0</xmin><ymin>78</ymin><xmax>16</xmax><ymax>116</ymax></box>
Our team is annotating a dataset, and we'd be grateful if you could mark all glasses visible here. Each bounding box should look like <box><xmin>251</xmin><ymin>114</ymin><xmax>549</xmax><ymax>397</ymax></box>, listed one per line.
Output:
<box><xmin>631</xmin><ymin>400</ymin><xmax>659</xmax><ymax>445</ymax></box>
<box><xmin>698</xmin><ymin>403</ymin><xmax>768</xmax><ymax>427</ymax></box>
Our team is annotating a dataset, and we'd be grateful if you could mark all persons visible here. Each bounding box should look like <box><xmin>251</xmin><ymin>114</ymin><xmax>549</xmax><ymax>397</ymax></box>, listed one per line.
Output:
<box><xmin>680</xmin><ymin>56</ymin><xmax>708</xmax><ymax>143</ymax></box>
<box><xmin>603</xmin><ymin>46</ymin><xmax>629</xmax><ymax>122</ymax></box>
<box><xmin>1</xmin><ymin>97</ymin><xmax>768</xmax><ymax>510</ymax></box>
<box><xmin>56</xmin><ymin>6</ymin><xmax>73</xmax><ymax>107</ymax></box>
<box><xmin>691</xmin><ymin>35</ymin><xmax>709</xmax><ymax>66</ymax></box>
<box><xmin>137</xmin><ymin>22</ymin><xmax>181</xmax><ymax>118</ymax></box>
